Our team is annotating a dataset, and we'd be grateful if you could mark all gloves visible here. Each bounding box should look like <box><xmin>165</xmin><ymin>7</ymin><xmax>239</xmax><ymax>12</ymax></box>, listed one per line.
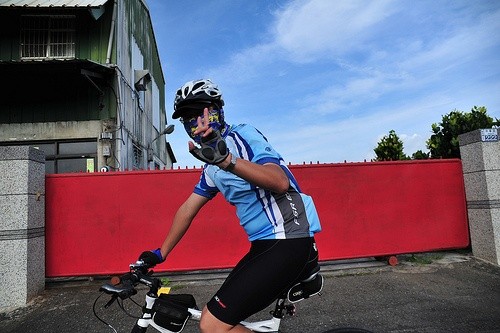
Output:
<box><xmin>139</xmin><ymin>250</ymin><xmax>159</xmax><ymax>268</ymax></box>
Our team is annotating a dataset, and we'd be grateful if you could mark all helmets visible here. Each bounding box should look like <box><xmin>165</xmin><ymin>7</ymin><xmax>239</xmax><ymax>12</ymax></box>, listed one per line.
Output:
<box><xmin>171</xmin><ymin>78</ymin><xmax>224</xmax><ymax>118</ymax></box>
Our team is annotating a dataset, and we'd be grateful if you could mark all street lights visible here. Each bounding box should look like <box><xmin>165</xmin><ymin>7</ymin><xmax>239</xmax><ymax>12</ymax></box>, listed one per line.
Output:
<box><xmin>141</xmin><ymin>124</ymin><xmax>175</xmax><ymax>149</ymax></box>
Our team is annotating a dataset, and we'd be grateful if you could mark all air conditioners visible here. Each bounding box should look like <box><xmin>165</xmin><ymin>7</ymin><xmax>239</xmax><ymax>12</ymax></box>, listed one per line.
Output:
<box><xmin>136</xmin><ymin>75</ymin><xmax>148</xmax><ymax>91</ymax></box>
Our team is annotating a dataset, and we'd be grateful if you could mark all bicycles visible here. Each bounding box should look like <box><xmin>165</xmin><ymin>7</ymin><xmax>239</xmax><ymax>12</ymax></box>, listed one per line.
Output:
<box><xmin>92</xmin><ymin>258</ymin><xmax>395</xmax><ymax>333</ymax></box>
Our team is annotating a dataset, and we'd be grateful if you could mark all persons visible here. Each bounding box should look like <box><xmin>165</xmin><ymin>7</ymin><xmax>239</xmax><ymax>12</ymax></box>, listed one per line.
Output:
<box><xmin>137</xmin><ymin>79</ymin><xmax>322</xmax><ymax>333</ymax></box>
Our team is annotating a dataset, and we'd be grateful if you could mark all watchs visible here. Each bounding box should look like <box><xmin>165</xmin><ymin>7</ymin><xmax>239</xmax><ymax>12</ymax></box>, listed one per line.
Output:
<box><xmin>225</xmin><ymin>154</ymin><xmax>236</xmax><ymax>172</ymax></box>
